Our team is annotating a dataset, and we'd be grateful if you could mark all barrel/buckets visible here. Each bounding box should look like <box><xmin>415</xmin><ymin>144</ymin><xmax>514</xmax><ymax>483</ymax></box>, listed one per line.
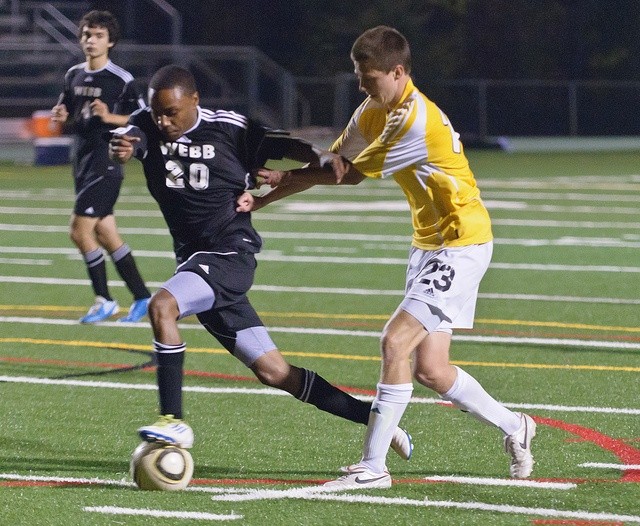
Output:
<box><xmin>34</xmin><ymin>137</ymin><xmax>75</xmax><ymax>163</ymax></box>
<box><xmin>31</xmin><ymin>109</ymin><xmax>61</xmax><ymax>136</ymax></box>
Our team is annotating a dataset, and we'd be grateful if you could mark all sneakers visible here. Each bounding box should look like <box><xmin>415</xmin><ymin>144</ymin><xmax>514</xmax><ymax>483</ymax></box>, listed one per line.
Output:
<box><xmin>137</xmin><ymin>414</ymin><xmax>195</xmax><ymax>448</ymax></box>
<box><xmin>390</xmin><ymin>425</ymin><xmax>413</xmax><ymax>460</ymax></box>
<box><xmin>81</xmin><ymin>298</ymin><xmax>119</xmax><ymax>325</ymax></box>
<box><xmin>505</xmin><ymin>412</ymin><xmax>537</xmax><ymax>479</ymax></box>
<box><xmin>123</xmin><ymin>298</ymin><xmax>152</xmax><ymax>320</ymax></box>
<box><xmin>323</xmin><ymin>468</ymin><xmax>391</xmax><ymax>492</ymax></box>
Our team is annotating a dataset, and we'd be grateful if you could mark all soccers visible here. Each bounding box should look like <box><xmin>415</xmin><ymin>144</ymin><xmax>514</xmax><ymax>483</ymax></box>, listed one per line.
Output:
<box><xmin>129</xmin><ymin>440</ymin><xmax>194</xmax><ymax>491</ymax></box>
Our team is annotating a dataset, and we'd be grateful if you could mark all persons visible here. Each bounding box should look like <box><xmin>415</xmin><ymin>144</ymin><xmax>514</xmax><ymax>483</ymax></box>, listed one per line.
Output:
<box><xmin>51</xmin><ymin>9</ymin><xmax>149</xmax><ymax>323</ymax></box>
<box><xmin>109</xmin><ymin>64</ymin><xmax>414</xmax><ymax>460</ymax></box>
<box><xmin>236</xmin><ymin>26</ymin><xmax>538</xmax><ymax>493</ymax></box>
